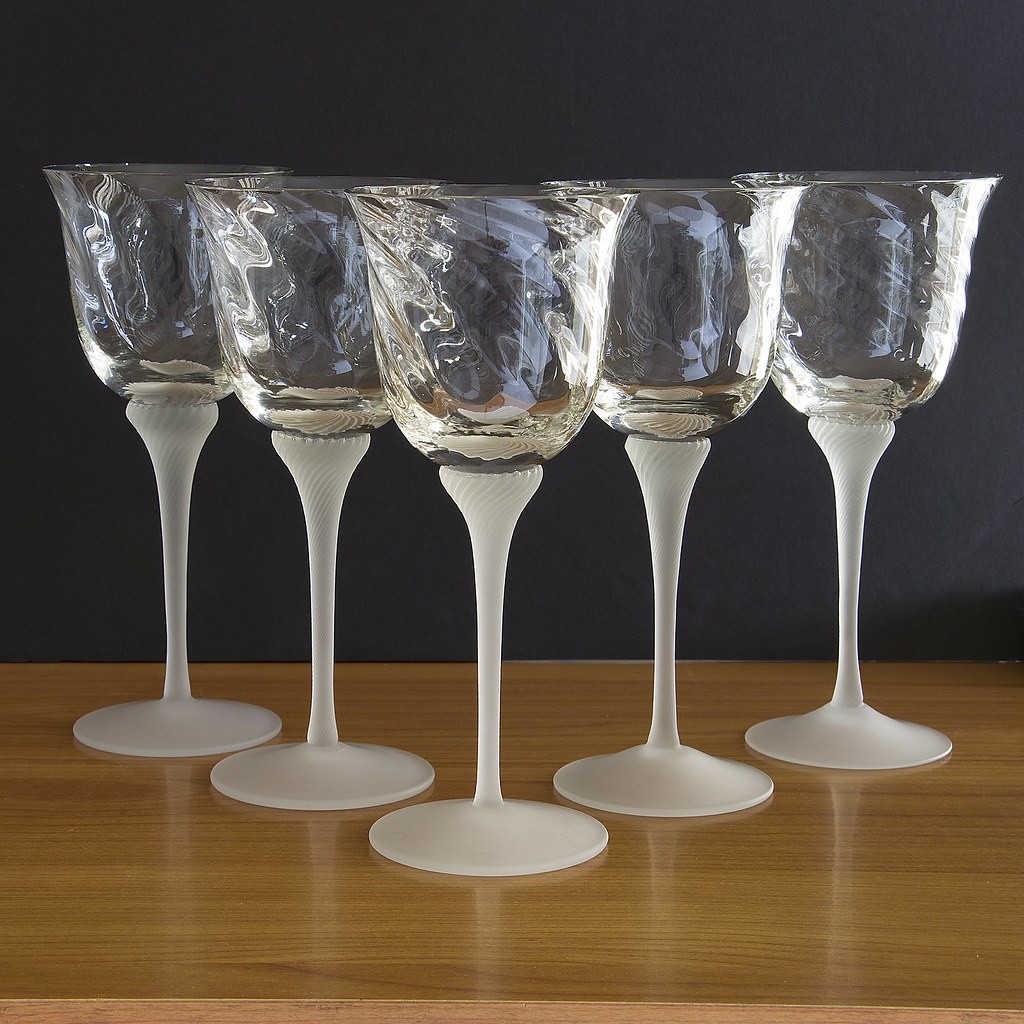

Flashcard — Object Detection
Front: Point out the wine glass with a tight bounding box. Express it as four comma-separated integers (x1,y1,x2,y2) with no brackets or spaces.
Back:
345,185,639,877
536,180,815,818
179,176,455,811
41,163,294,760
732,170,1004,771
